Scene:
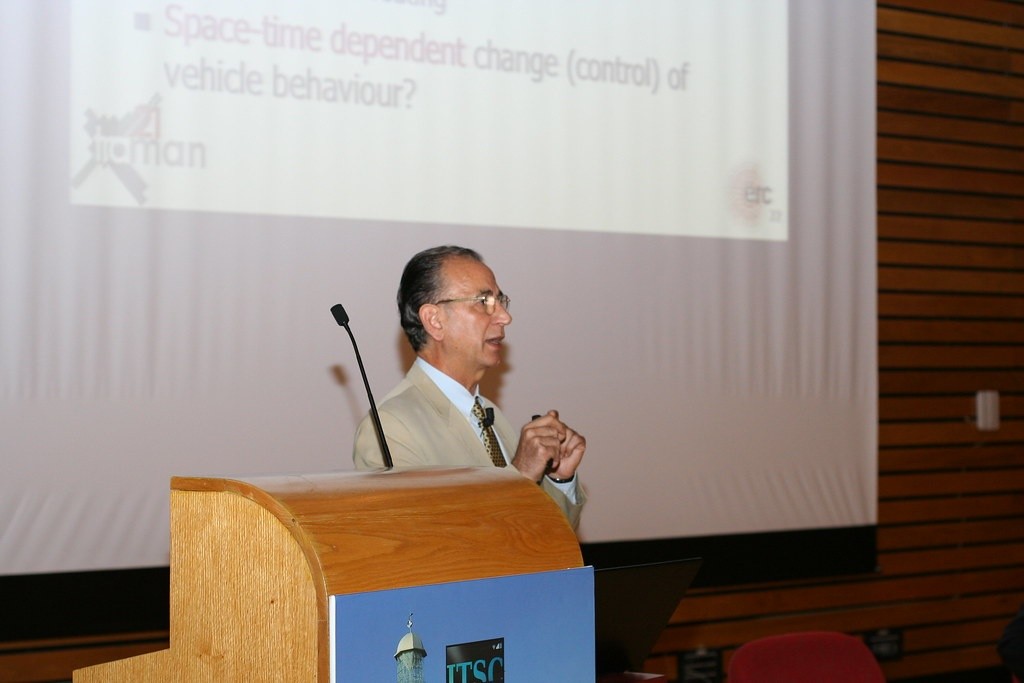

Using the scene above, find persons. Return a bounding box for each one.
[353,242,588,539]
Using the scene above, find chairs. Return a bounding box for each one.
[725,632,888,683]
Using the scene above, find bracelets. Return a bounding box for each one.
[546,473,576,483]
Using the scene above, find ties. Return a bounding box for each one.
[471,398,507,467]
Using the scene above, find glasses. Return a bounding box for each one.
[436,293,512,315]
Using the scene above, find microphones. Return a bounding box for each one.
[329,304,394,470]
[482,408,494,427]
